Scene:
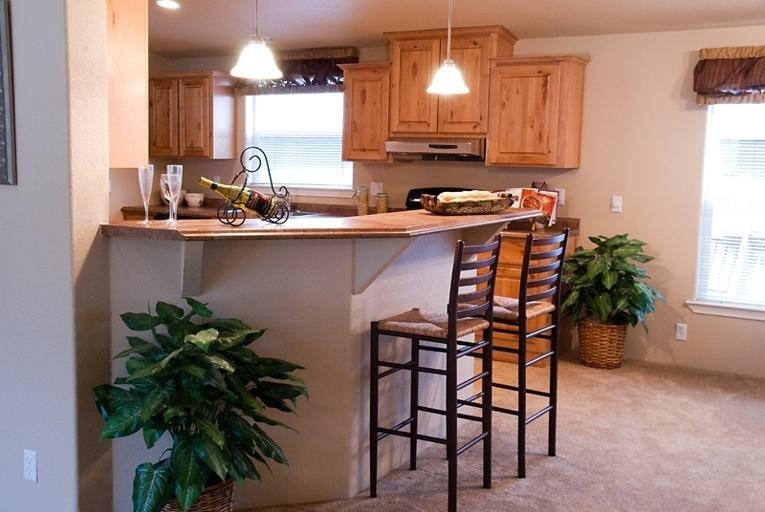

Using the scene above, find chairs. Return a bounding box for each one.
[370,234,502,511]
[447,227,569,478]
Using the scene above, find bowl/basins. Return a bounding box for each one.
[185,193,204,207]
[162,191,185,206]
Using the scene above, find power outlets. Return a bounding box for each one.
[23,449,37,482]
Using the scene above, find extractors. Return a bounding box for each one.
[385,139,489,162]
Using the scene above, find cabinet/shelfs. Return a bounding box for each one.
[486,55,590,169]
[336,61,393,162]
[149,71,236,161]
[476,232,546,369]
[383,25,520,139]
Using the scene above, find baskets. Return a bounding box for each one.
[420,192,514,215]
[577,312,629,370]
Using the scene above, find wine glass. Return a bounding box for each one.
[168,163,182,230]
[160,173,178,226]
[137,165,155,226]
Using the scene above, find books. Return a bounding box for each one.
[494,187,560,227]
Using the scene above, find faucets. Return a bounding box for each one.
[276,192,291,210]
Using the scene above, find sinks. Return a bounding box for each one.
[290,212,312,216]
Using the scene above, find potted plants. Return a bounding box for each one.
[562,234,665,369]
[91,292,309,512]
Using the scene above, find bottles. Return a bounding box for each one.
[356,187,368,217]
[198,176,280,220]
[377,192,389,214]
